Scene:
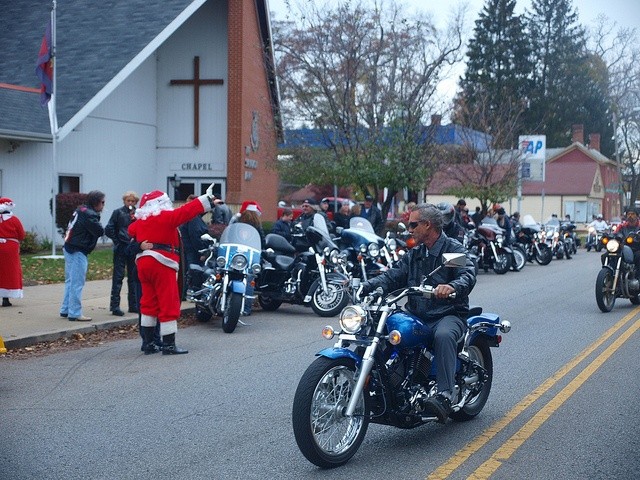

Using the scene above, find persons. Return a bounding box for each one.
[228,200,267,316]
[127,190,217,355]
[104,191,140,316]
[587,213,611,233]
[497,207,520,272]
[334,200,349,228]
[461,208,475,226]
[211,197,232,226]
[124,236,163,351]
[179,194,224,300]
[450,199,467,238]
[401,202,416,222]
[294,198,329,238]
[612,208,640,296]
[472,207,482,226]
[509,212,524,234]
[435,202,465,245]
[320,197,333,221]
[341,203,477,424]
[610,224,617,233]
[486,204,500,220]
[559,214,577,254]
[272,208,293,243]
[0,198,27,307]
[59,191,106,321]
[349,205,361,217]
[592,214,598,221]
[544,213,560,246]
[358,194,385,234]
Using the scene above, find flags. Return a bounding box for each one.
[35,17,54,111]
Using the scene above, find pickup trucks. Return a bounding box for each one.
[277,196,356,221]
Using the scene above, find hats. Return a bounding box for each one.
[237,199,264,218]
[493,204,502,211]
[302,199,316,206]
[626,206,638,216]
[366,195,373,200]
[498,207,505,214]
[0,198,16,212]
[135,190,168,214]
[320,198,330,202]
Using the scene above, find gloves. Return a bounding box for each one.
[206,182,215,196]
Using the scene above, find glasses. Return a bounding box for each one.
[102,201,105,203]
[408,220,424,228]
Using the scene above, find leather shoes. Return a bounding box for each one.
[60,314,68,317]
[69,314,93,321]
[112,310,124,316]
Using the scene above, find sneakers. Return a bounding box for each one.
[426,394,455,424]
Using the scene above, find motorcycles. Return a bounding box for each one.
[333,216,392,284]
[457,215,513,275]
[379,231,411,266]
[292,247,513,469]
[554,221,582,260]
[595,229,639,313]
[542,217,564,261]
[583,224,606,252]
[183,221,263,333]
[513,214,552,265]
[510,242,527,271]
[257,212,351,318]
[396,222,416,249]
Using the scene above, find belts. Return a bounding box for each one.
[152,242,181,255]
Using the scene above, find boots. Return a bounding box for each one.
[144,327,159,355]
[162,333,189,355]
